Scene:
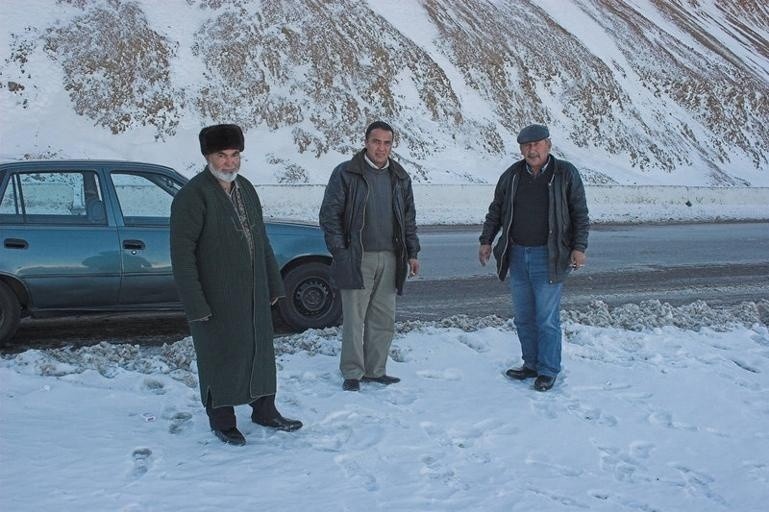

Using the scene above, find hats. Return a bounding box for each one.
[516,123,550,144]
[198,123,245,154]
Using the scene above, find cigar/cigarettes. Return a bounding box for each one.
[569,264,585,268]
[407,272,416,278]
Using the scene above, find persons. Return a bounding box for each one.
[318,121,422,393]
[478,123,592,392]
[168,123,304,448]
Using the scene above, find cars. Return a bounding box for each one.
[0,161,347,345]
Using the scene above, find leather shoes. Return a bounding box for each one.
[342,378,361,392]
[252,411,303,431]
[506,366,537,380]
[211,425,246,446]
[365,374,400,385]
[534,374,557,391]
